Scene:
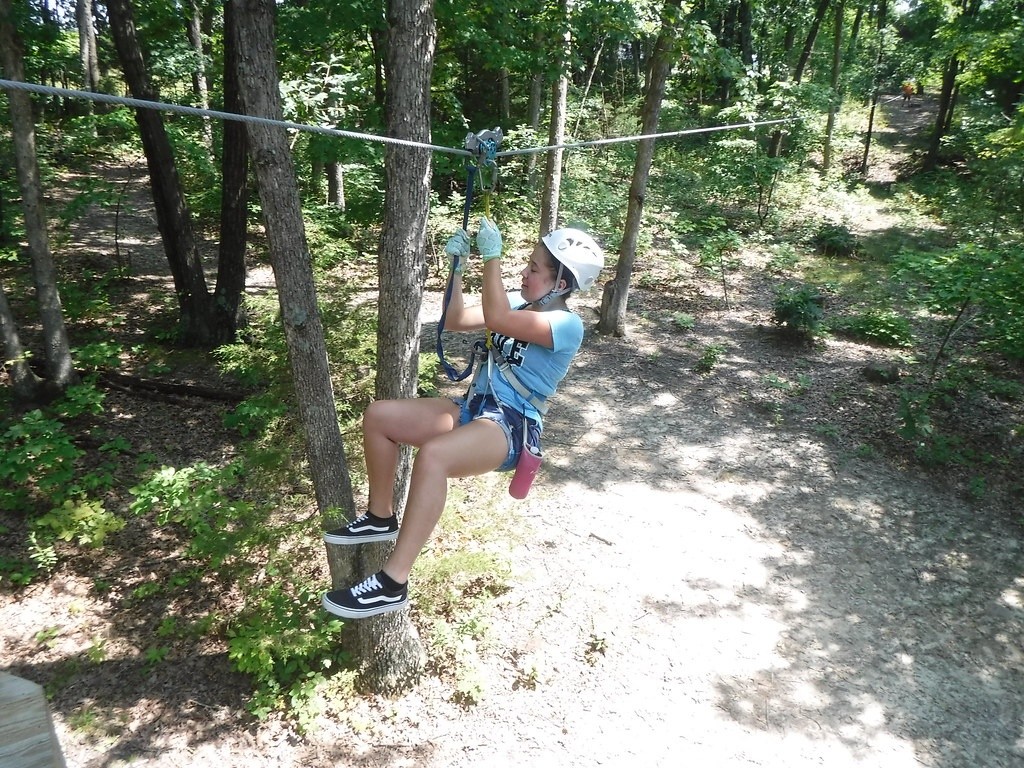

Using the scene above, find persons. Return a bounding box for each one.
[320,216,604,619]
[901,82,913,109]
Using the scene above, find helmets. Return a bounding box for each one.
[542,228,604,291]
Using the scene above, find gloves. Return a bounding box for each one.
[444,229,471,273]
[476,215,502,263]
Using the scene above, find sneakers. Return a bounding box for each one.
[323,511,399,545]
[321,571,408,619]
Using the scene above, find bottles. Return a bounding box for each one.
[529,446,540,456]
